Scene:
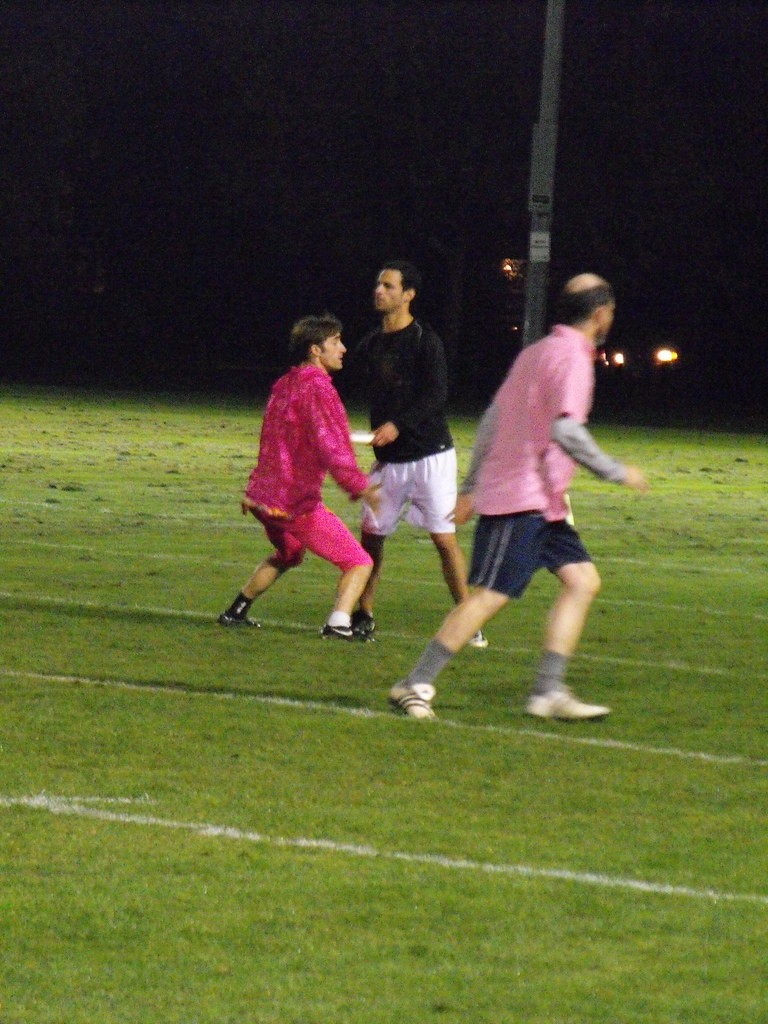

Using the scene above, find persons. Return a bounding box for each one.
[388,272,652,720]
[215,259,490,650]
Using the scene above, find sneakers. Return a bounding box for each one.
[526,682,612,719]
[319,624,371,644]
[389,682,437,722]
[216,609,262,628]
[351,609,376,635]
[470,630,490,648]
